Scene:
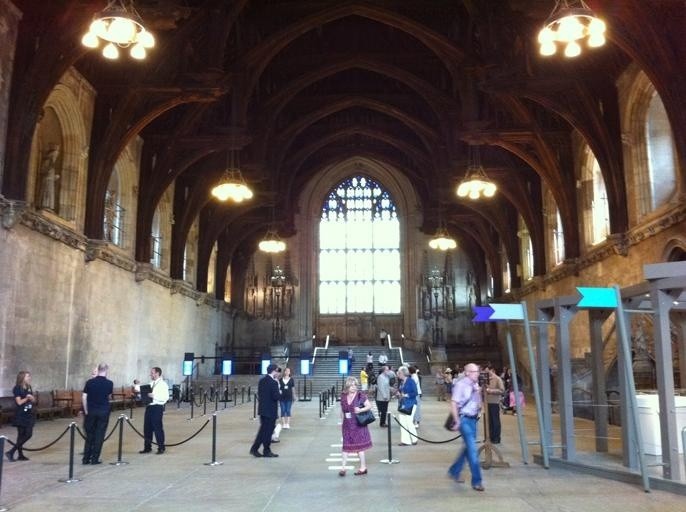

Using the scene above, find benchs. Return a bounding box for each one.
[0,383,144,422]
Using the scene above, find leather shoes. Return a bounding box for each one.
[398,442,411,446]
[411,442,418,445]
[338,469,346,476]
[154,447,166,456]
[262,452,280,457]
[353,467,369,477]
[414,424,419,430]
[472,483,485,492]
[80,457,104,465]
[446,471,466,484]
[138,448,152,453]
[248,447,263,458]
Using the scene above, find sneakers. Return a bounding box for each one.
[270,438,281,443]
[16,456,29,462]
[379,423,389,428]
[4,450,16,463]
[281,423,290,429]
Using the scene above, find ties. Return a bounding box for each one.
[151,381,156,390]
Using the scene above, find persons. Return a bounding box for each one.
[432,364,523,417]
[448,362,486,493]
[132,379,145,407]
[346,347,353,371]
[269,367,283,443]
[3,371,37,463]
[379,328,387,346]
[81,363,112,466]
[278,367,296,430]
[481,362,506,443]
[137,366,168,455]
[249,364,283,458]
[337,375,372,477]
[359,350,422,447]
[79,367,98,455]
[135,380,141,399]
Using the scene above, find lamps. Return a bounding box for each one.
[453,138,498,199]
[429,198,457,252]
[534,0,610,58]
[259,191,287,254]
[79,0,155,62]
[208,83,255,203]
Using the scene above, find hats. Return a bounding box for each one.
[445,367,453,372]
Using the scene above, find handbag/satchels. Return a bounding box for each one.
[396,395,415,416]
[354,391,376,426]
[443,406,465,432]
